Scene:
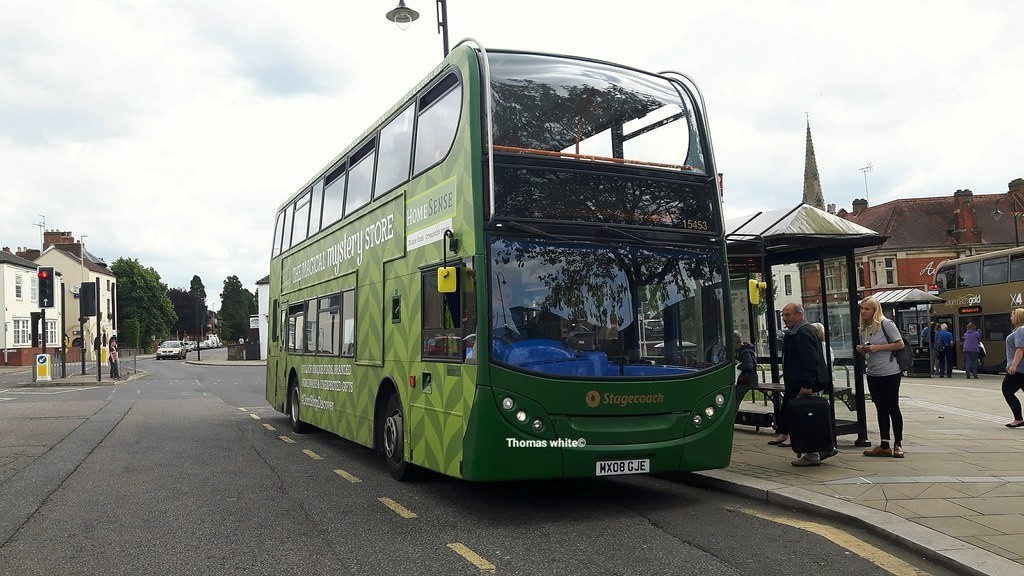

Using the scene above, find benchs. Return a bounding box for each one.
[751,365,855,412]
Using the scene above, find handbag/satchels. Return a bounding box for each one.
[935,338,945,352]
[733,362,742,387]
[978,342,987,357]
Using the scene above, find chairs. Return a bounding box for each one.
[514,143,561,158]
[536,307,564,343]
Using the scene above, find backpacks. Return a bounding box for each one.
[880,320,913,370]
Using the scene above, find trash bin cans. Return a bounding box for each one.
[246,340,259,360]
[907,347,931,378]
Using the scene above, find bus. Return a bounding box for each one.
[265,37,738,484]
[931,246,1024,374]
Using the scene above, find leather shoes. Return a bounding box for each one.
[863,446,892,456]
[892,445,904,458]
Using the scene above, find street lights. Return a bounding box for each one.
[386,0,449,59]
[990,197,1019,247]
[81,235,88,282]
[195,299,200,360]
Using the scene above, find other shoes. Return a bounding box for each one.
[791,454,821,467]
[947,375,952,378]
[939,374,944,378]
[1005,420,1024,427]
[768,437,791,447]
[821,447,839,460]
[974,376,979,379]
[967,376,970,379]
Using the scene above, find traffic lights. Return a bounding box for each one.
[212,303,216,333]
[38,267,53,307]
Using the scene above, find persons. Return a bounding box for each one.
[1002,308,1024,428]
[491,283,519,335]
[963,322,981,379]
[767,322,835,447]
[109,339,120,380]
[856,297,906,458]
[782,303,839,467]
[920,321,955,378]
[733,330,758,421]
[238,336,244,345]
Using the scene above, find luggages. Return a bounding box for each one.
[790,395,838,458]
